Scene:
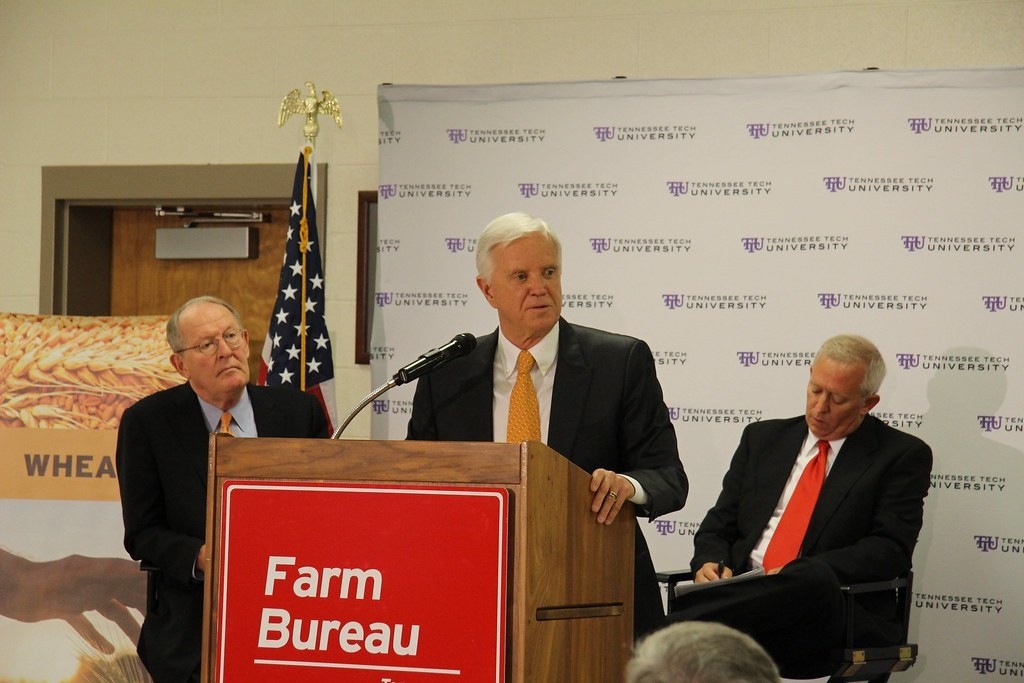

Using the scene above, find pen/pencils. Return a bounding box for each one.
[718,564,721,579]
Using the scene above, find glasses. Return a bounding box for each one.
[176,328,243,356]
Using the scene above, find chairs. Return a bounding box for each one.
[656,567,918,683]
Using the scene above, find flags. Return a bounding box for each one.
[255,142,338,436]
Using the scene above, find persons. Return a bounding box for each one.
[690,335,933,585]
[116,296,331,683]
[405,212,690,643]
[626,621,778,683]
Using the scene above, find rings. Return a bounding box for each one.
[609,491,617,499]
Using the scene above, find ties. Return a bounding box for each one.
[219,412,233,433]
[762,440,830,576]
[506,351,541,442]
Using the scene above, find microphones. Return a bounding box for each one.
[398,333,477,383]
